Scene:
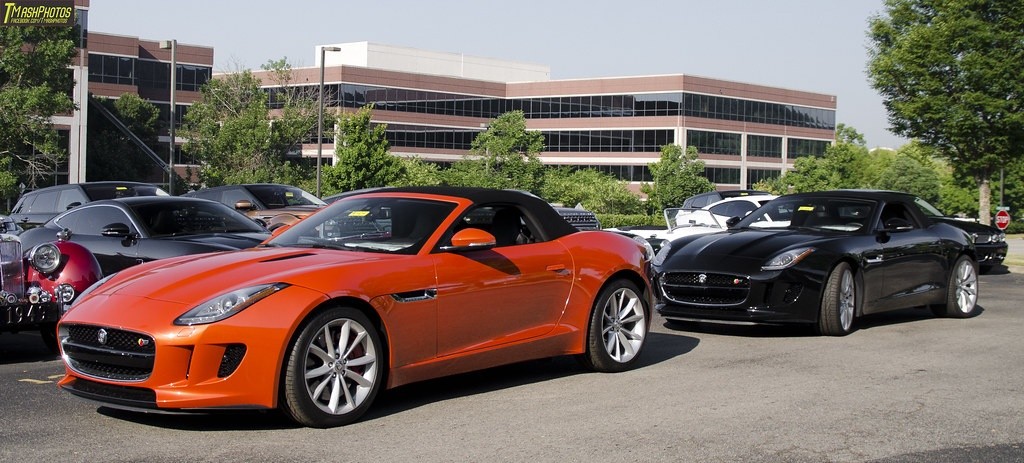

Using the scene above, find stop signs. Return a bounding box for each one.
[995,210,1010,230]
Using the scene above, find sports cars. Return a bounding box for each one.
[59,186,652,428]
[650,189,981,336]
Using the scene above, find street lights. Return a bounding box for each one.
[160,39,178,195]
[317,46,341,198]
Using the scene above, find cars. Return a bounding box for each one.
[912,196,1008,274]
[178,182,330,232]
[676,195,791,227]
[0,217,106,354]
[603,207,729,254]
[12,181,171,237]
[551,203,600,234]
[677,190,775,216]
[18,195,272,277]
